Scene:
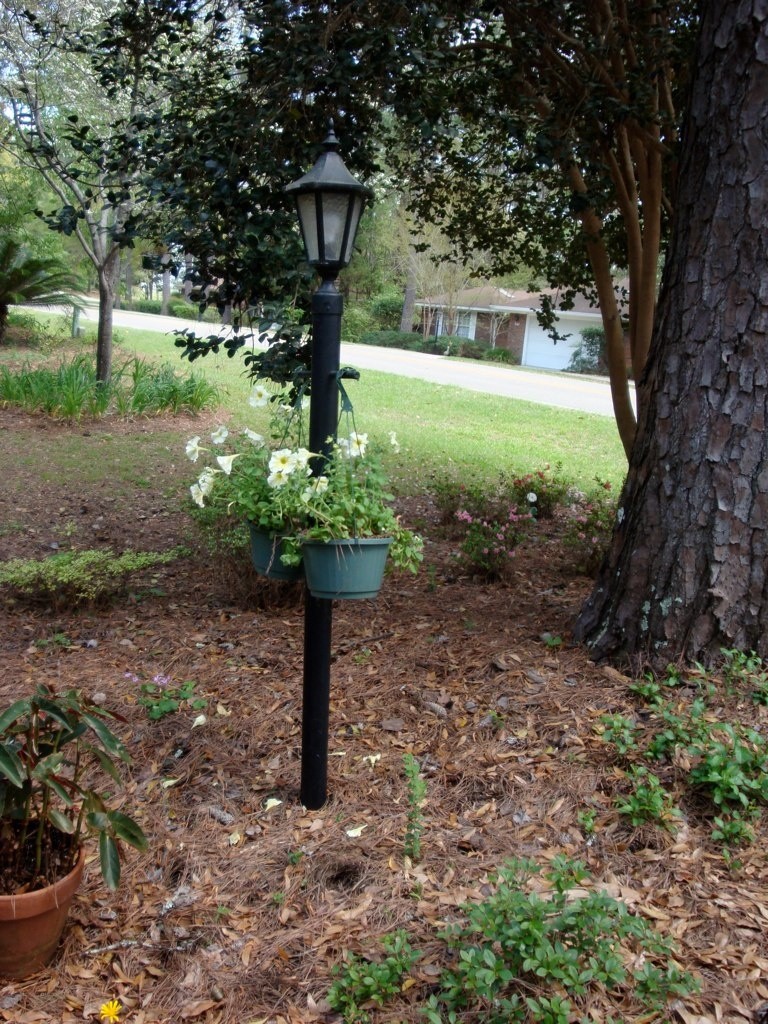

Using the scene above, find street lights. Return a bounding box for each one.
[279,128,375,811]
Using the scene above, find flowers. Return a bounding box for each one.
[186,390,313,534]
[269,433,424,575]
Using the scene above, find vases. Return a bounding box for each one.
[240,516,299,580]
[299,537,393,599]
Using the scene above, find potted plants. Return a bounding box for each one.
[0,680,148,978]
